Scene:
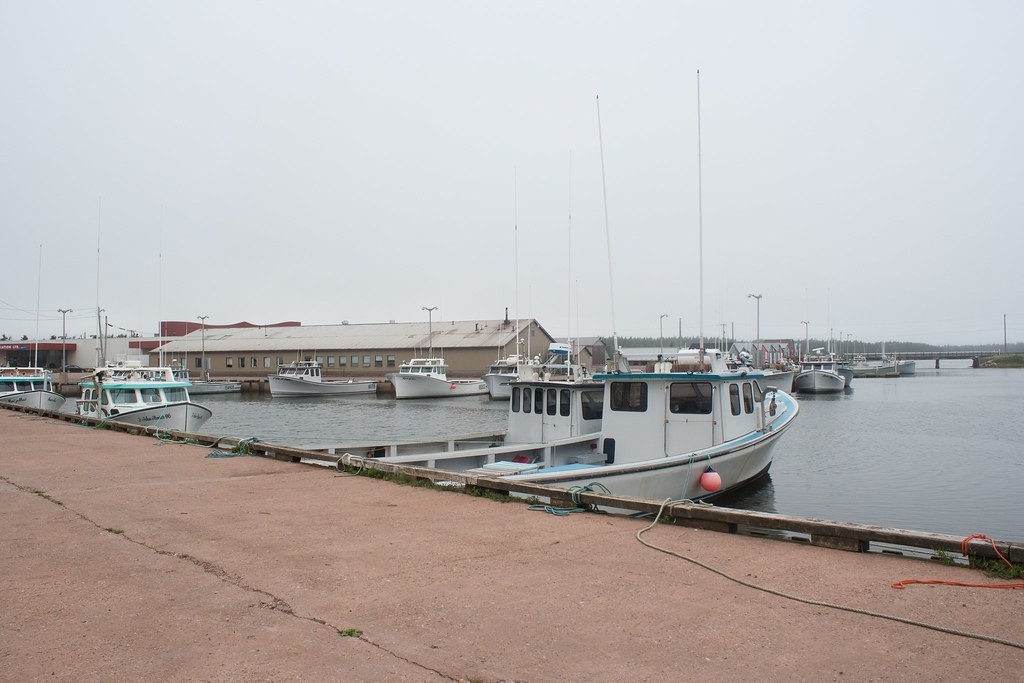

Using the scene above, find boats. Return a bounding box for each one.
[298,370,800,518]
[386,341,489,400]
[267,341,377,399]
[0,189,244,437]
[481,66,915,401]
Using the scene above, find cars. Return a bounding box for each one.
[60,364,86,373]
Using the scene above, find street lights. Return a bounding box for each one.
[748,293,762,369]
[660,314,668,353]
[57,308,73,373]
[800,321,811,354]
[197,315,208,369]
[421,307,438,358]
